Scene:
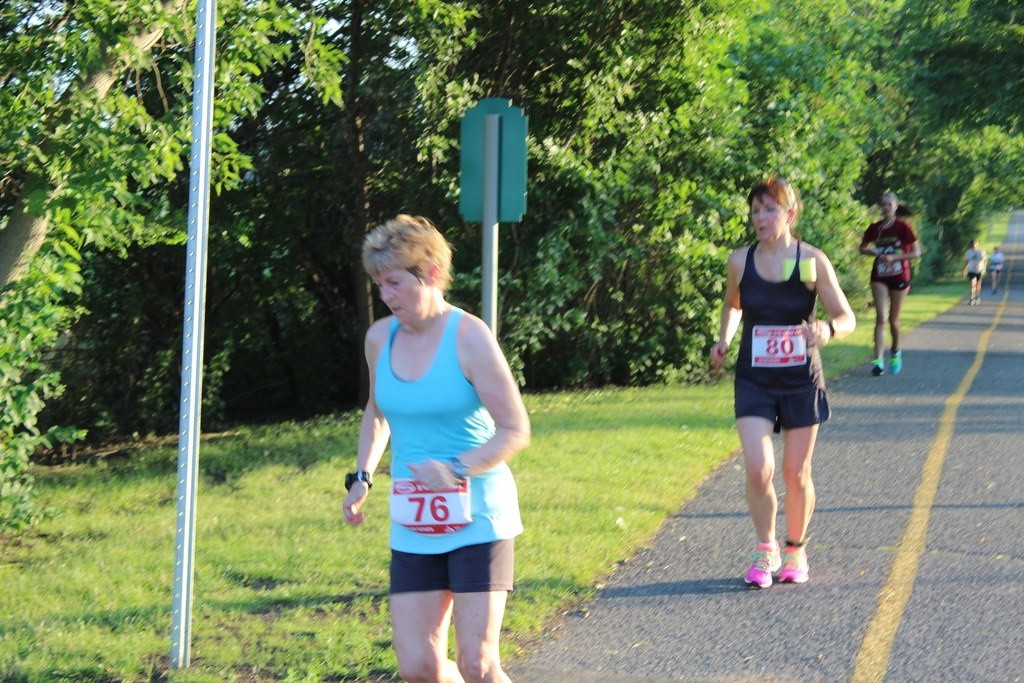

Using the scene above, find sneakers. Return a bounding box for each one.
[744,543,781,590]
[889,350,903,375]
[778,546,811,586]
[871,356,885,377]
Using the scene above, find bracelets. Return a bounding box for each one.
[447,456,469,478]
[709,174,857,594]
[827,319,837,338]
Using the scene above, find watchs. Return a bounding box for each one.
[344,470,375,493]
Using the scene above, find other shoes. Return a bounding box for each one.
[968,296,980,306]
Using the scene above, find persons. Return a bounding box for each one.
[855,188,925,379]
[342,213,533,683]
[988,245,1006,296]
[961,237,985,307]
